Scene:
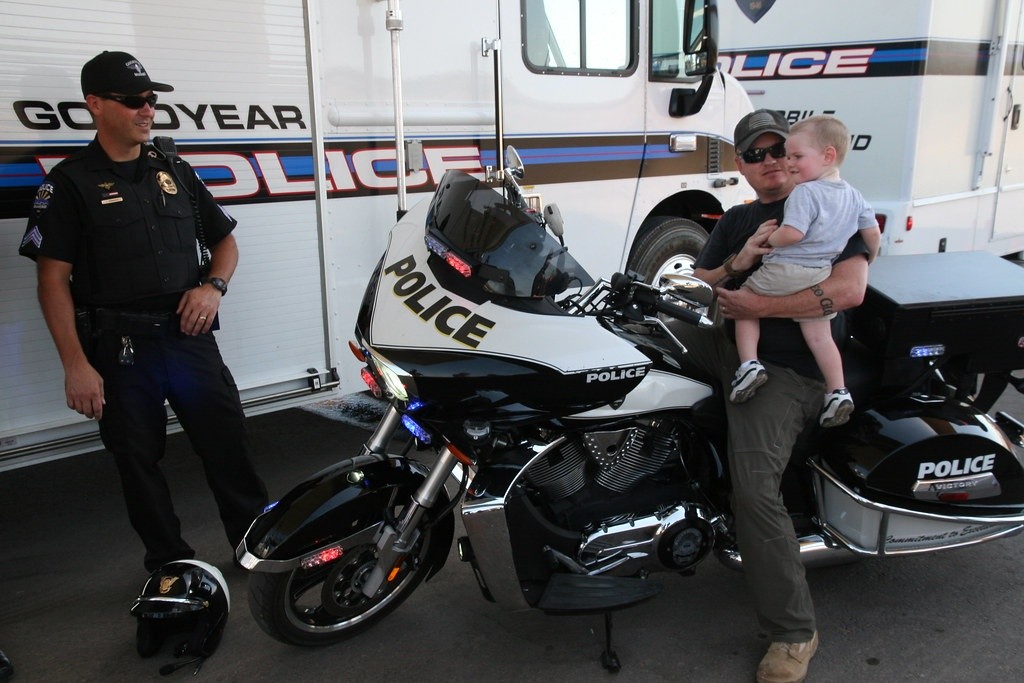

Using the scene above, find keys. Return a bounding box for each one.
[118,335,136,367]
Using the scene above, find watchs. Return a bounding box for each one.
[722,252,742,277]
[201,276,227,297]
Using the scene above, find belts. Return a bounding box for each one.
[75,304,179,337]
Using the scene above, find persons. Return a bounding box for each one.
[690,109,881,683]
[18,50,271,573]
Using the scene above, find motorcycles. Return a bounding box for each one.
[236,147,1024,676]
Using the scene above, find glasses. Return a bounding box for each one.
[98,94,157,109]
[736,141,786,164]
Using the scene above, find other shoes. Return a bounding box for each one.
[756,630,818,683]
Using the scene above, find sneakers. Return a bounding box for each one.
[819,387,854,427]
[728,360,768,403]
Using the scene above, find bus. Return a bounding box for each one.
[651,1,1024,279]
[0,2,772,474]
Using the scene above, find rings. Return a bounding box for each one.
[198,316,207,320]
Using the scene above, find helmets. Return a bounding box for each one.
[130,558,230,659]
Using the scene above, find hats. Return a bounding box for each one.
[734,108,790,155]
[81,50,174,100]
